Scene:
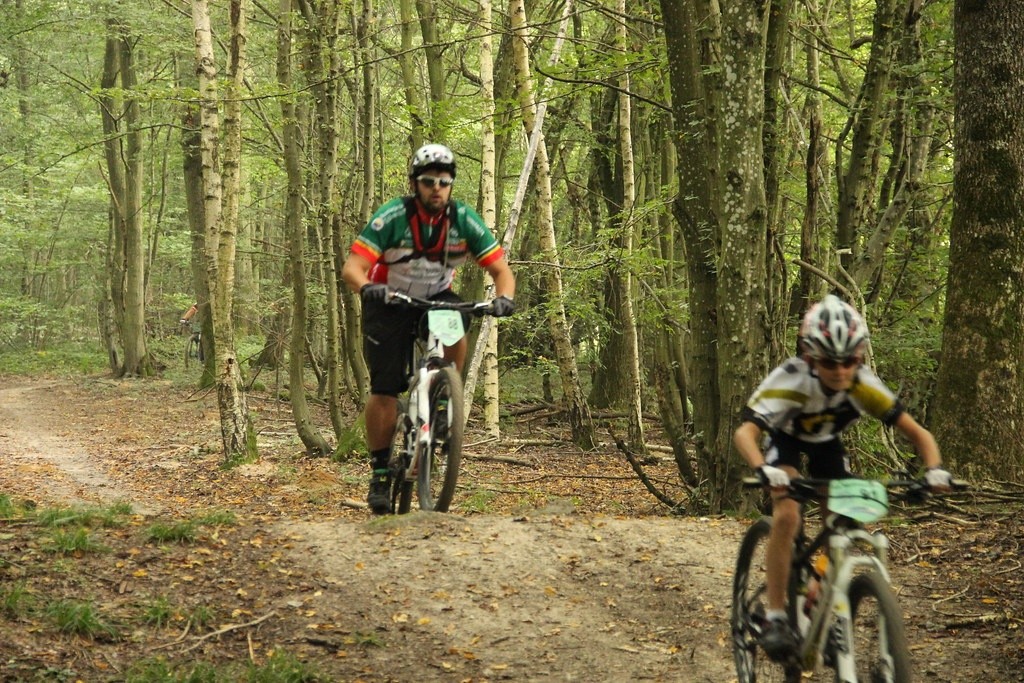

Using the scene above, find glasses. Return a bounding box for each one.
[417,175,453,188]
[813,357,860,370]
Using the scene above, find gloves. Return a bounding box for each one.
[925,469,951,492]
[492,296,516,317]
[360,284,390,305]
[755,465,790,487]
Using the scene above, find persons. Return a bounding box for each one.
[342,143,516,513]
[732,294,951,656]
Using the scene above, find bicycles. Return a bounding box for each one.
[388,290,496,514]
[730,476,971,683]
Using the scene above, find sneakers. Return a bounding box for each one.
[435,400,448,439]
[367,467,391,513]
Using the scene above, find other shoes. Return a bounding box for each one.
[763,611,798,662]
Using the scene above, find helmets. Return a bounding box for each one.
[797,288,869,360]
[409,144,457,178]
